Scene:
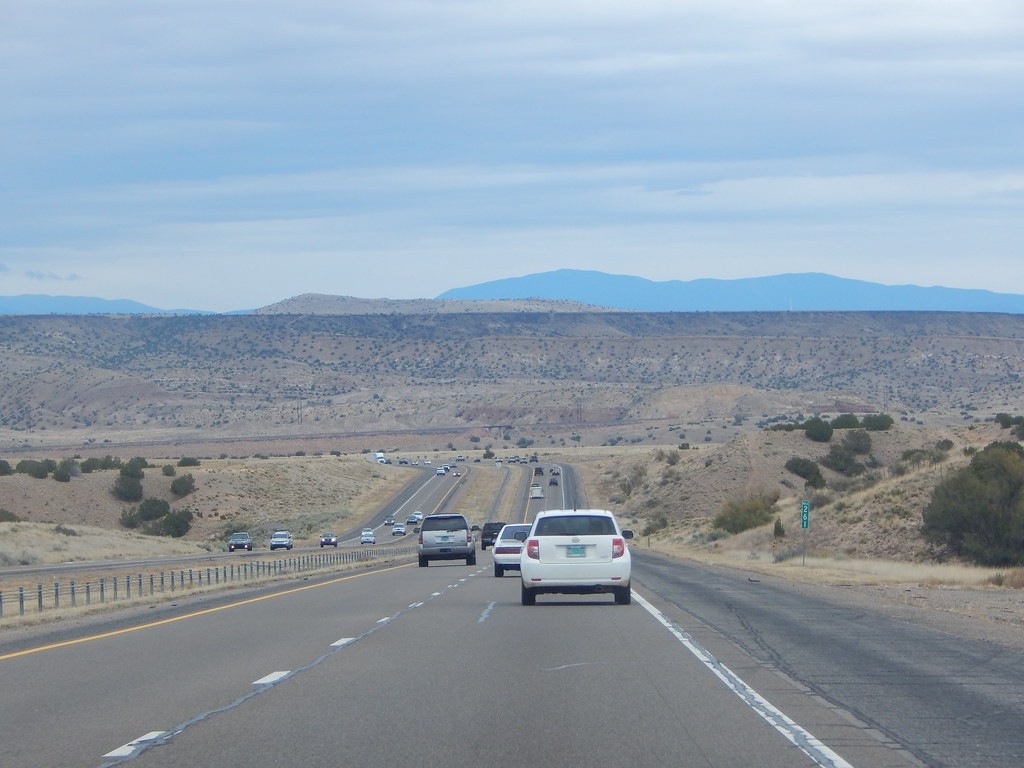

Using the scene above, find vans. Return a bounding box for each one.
[529,486,544,499]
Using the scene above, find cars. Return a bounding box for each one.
[384,458,392,463]
[384,509,423,537]
[436,455,466,476]
[359,526,377,546]
[534,467,545,476]
[494,454,538,464]
[548,465,561,487]
[423,459,432,465]
[472,456,483,462]
[228,531,256,551]
[319,531,337,546]
[411,459,419,465]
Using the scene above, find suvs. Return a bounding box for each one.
[399,457,408,465]
[481,521,533,577]
[513,509,633,607]
[416,512,480,567]
[270,529,294,550]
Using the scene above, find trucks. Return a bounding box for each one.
[373,453,386,463]
